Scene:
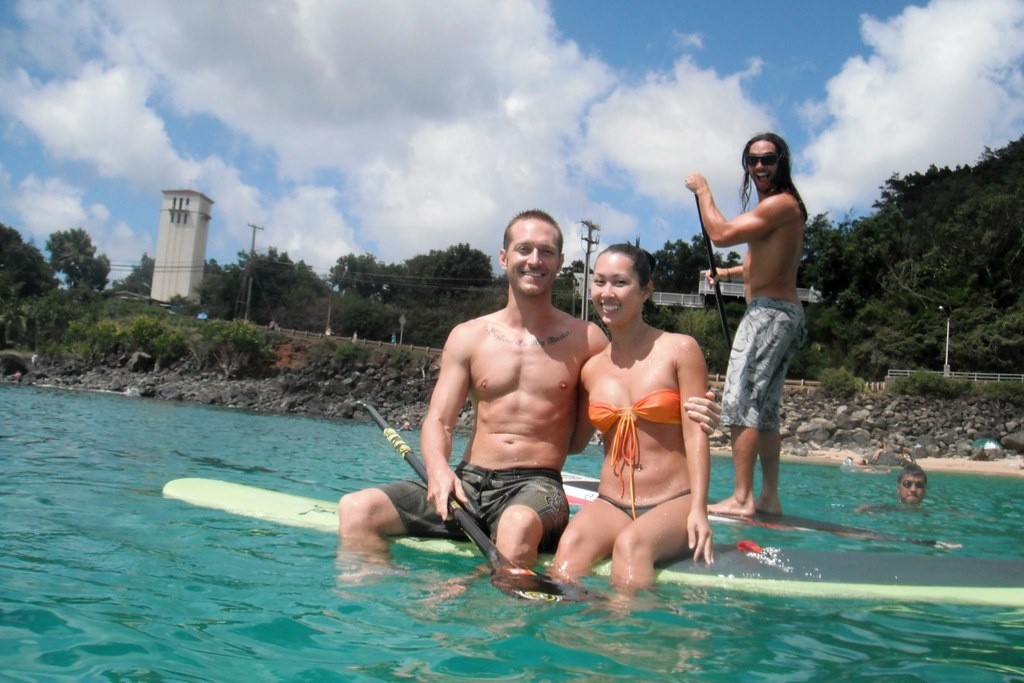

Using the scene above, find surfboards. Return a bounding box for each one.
[559,470,963,550]
[159,475,1023,610]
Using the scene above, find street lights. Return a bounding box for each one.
[938,305,950,377]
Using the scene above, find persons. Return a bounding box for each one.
[338,211,721,583]
[683,132,808,517]
[897,464,927,504]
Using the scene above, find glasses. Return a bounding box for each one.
[745,154,779,167]
[899,480,925,489]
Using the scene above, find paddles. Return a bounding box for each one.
[693,191,733,349]
[359,400,523,568]
